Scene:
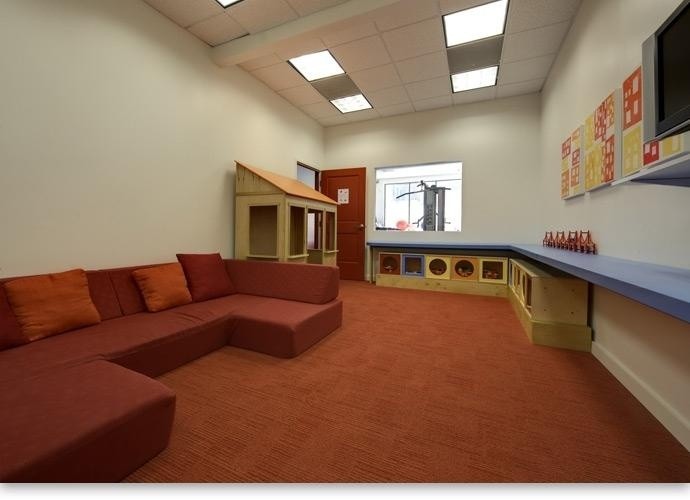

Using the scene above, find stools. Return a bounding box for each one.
[1,356,179,483]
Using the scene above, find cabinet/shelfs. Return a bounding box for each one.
[373,249,509,301]
[504,255,594,355]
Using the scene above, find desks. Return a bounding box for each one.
[364,237,690,327]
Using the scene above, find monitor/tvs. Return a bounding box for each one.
[641,0,690,145]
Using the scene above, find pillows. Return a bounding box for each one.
[130,262,192,314]
[4,266,101,345]
[175,251,242,304]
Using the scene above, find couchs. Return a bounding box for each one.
[0,256,345,382]
